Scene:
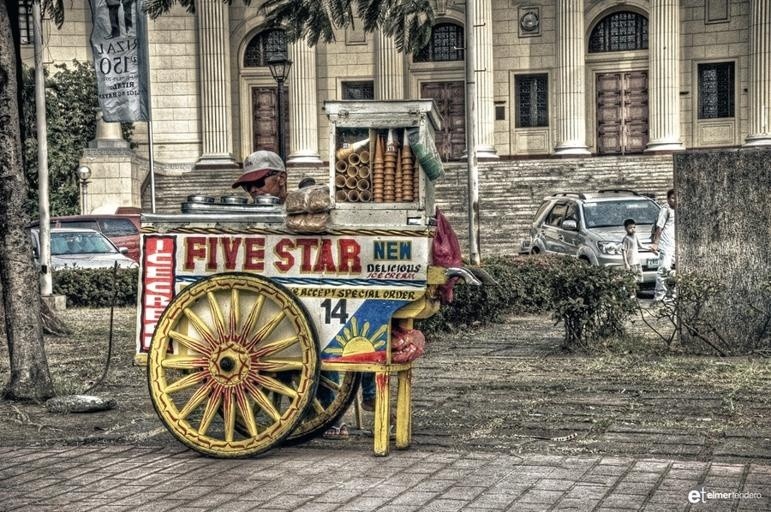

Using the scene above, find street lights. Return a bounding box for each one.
[266,48,295,194]
[75,165,92,217]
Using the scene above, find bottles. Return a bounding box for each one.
[410,140,441,179]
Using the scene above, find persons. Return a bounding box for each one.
[621,219,652,306]
[232,150,396,440]
[652,188,675,307]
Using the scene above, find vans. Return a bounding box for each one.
[28,214,141,265]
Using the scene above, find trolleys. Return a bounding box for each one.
[137,95,445,459]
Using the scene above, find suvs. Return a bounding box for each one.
[529,185,680,299]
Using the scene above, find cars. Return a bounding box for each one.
[27,226,140,273]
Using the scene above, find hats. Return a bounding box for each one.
[232,149,286,192]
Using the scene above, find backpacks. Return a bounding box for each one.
[649,210,669,242]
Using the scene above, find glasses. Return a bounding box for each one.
[241,176,271,192]
